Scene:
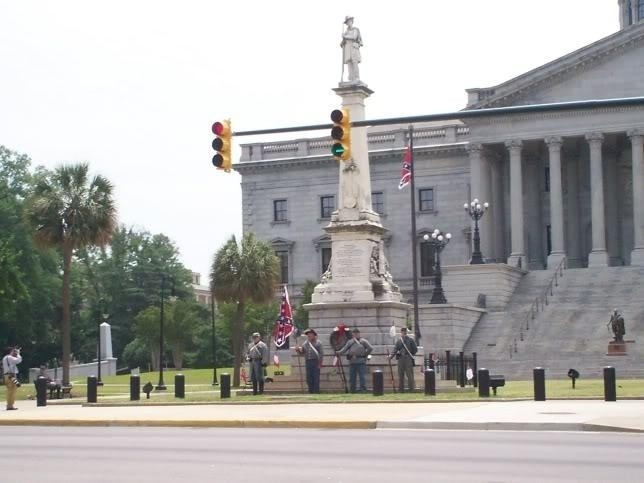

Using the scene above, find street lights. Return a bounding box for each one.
[209,272,219,385]
[423,229,452,305]
[463,197,489,265]
[155,276,179,390]
[96,297,110,386]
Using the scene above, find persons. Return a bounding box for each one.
[387,327,418,392]
[606,308,625,344]
[2,347,22,410]
[340,16,364,82]
[245,333,268,395]
[33,363,62,399]
[295,328,324,393]
[335,328,374,393]
[432,352,441,373]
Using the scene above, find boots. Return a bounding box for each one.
[253,380,257,394]
[258,382,263,393]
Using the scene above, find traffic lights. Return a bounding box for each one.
[211,121,232,171]
[330,108,352,161]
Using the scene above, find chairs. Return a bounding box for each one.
[38,375,55,399]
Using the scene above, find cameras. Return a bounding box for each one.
[14,348,17,353]
[8,375,21,387]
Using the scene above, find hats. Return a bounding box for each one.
[352,329,360,333]
[253,332,260,336]
[305,329,317,336]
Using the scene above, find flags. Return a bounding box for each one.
[274,289,294,348]
[398,139,412,190]
